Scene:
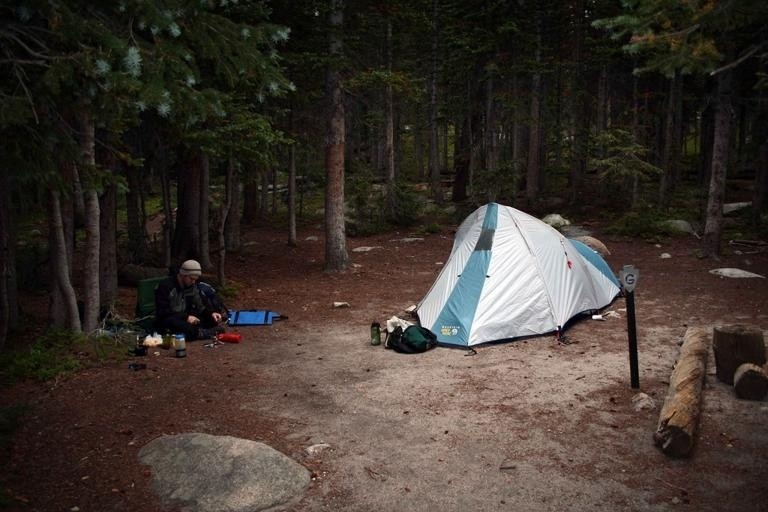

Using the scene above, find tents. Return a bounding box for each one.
[404,199,620,350]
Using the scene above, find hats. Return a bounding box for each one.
[180,259,201,276]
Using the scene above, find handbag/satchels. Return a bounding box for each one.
[400,325,438,352]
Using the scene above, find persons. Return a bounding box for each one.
[154,260,232,343]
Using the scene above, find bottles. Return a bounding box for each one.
[370,319,381,346]
[162,332,186,358]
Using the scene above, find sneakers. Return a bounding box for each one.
[205,326,225,336]
